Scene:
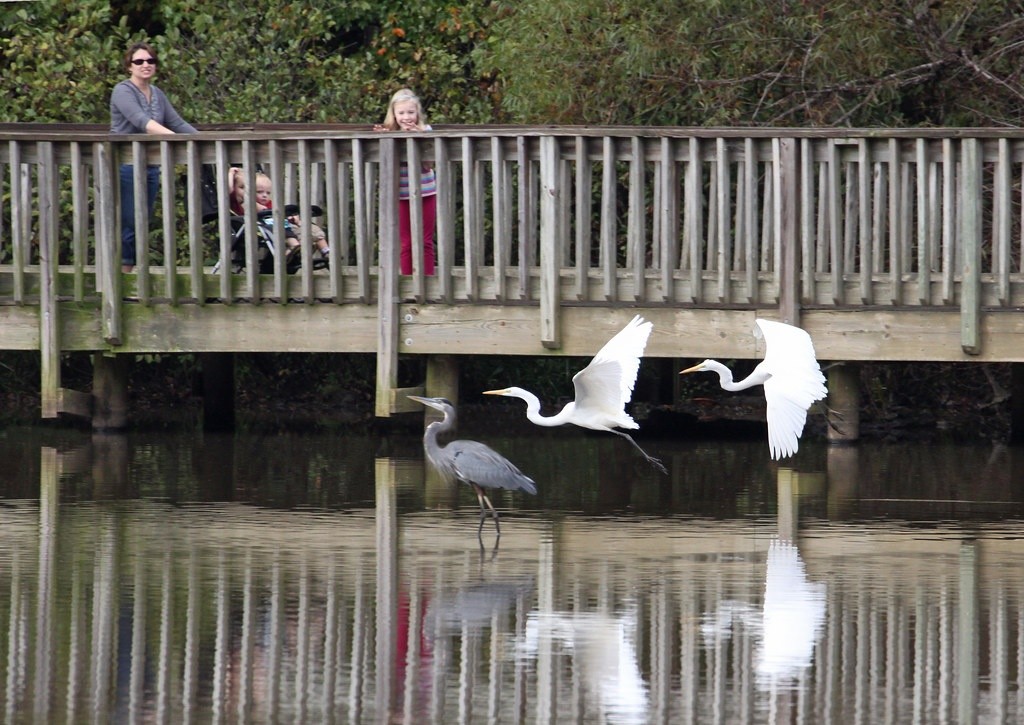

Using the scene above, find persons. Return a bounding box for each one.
[253,169,336,266]
[227,165,306,268]
[374,87,439,277]
[108,40,202,304]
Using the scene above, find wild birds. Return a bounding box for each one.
[403,390,538,536]
[481,312,668,479]
[677,318,848,461]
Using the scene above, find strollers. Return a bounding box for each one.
[185,163,325,275]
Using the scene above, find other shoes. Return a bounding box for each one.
[322,250,329,258]
[286,245,301,266]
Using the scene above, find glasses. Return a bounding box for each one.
[130,58,155,65]
